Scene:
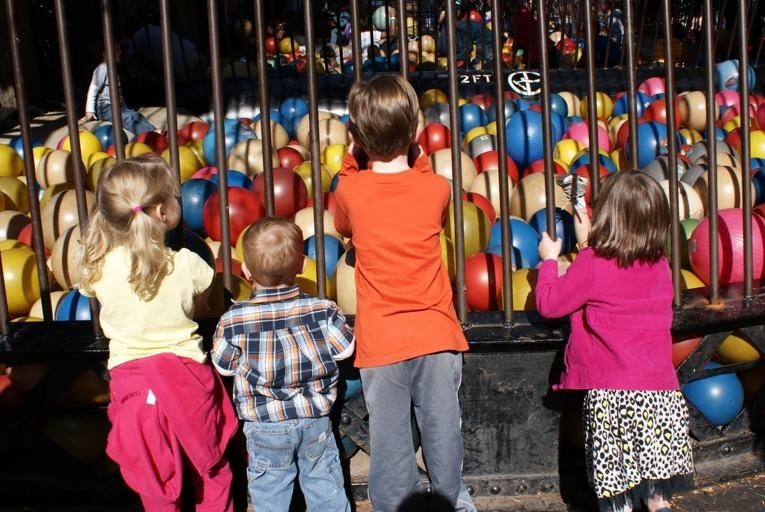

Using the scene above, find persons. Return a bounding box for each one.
[211,219,357,512]
[264,2,765,93]
[536,170,695,512]
[79,159,239,512]
[77,36,168,135]
[334,74,474,512]
